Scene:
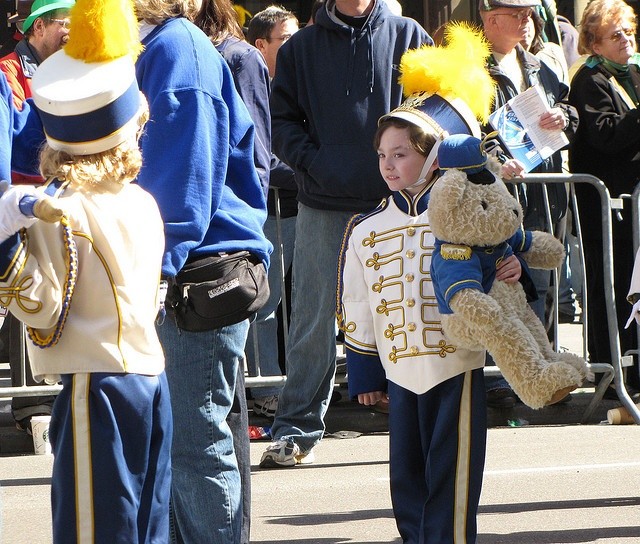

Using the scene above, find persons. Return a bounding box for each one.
[0,0,76,197]
[519,10,570,105]
[570,0,640,402]
[131,0,275,543]
[245,4,299,419]
[336,19,522,544]
[194,0,272,202]
[259,0,436,466]
[0,0,173,544]
[478,0,580,330]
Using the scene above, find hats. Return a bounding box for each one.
[378,90,498,190]
[29,46,149,156]
[478,0,541,11]
[7,1,33,23]
[22,0,76,32]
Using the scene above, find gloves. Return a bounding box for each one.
[624,300,640,329]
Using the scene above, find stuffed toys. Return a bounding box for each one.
[428,133,587,411]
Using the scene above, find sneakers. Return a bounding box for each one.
[259,436,315,468]
[15,413,51,436]
[252,395,278,422]
[485,388,517,408]
[351,395,389,414]
[595,384,640,404]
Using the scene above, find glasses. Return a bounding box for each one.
[50,18,71,30]
[494,8,532,20]
[602,28,634,42]
[261,35,291,41]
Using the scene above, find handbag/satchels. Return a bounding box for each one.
[164,250,271,332]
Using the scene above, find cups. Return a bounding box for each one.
[606,403,640,424]
